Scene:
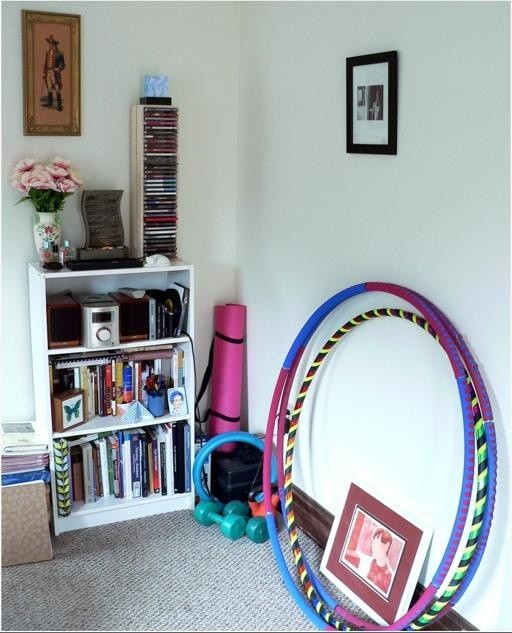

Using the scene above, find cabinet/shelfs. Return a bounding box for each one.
[28,262,196,538]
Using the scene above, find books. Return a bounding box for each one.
[142,107,179,261]
[2,343,195,517]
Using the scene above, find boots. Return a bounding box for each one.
[57,94,63,111]
[42,93,52,107]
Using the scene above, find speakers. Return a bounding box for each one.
[46,294,149,349]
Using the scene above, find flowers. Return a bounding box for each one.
[10,156,83,211]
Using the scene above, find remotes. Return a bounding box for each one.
[117,286,146,299]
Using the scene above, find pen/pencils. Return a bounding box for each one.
[146,367,167,390]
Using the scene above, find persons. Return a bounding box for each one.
[369,85,383,119]
[41,34,67,114]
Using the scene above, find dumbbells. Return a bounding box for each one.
[222,499,270,544]
[191,499,247,542]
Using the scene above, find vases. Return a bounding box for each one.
[34,212,61,264]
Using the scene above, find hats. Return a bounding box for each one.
[45,34,59,45]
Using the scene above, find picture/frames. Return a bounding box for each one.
[318,476,435,626]
[346,50,399,153]
[21,8,81,137]
